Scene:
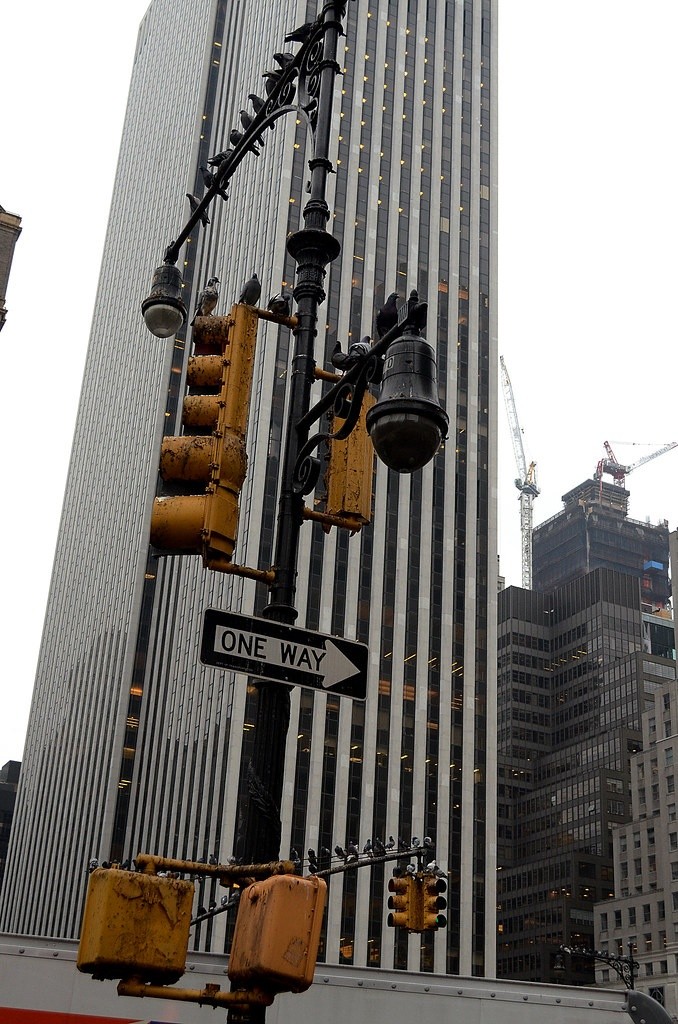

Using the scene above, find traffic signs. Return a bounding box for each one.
[195,607,371,703]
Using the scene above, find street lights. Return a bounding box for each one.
[74,2,457,1023]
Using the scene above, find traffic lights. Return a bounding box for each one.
[423,877,454,933]
[316,387,377,533]
[384,876,410,935]
[146,301,260,578]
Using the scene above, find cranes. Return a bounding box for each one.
[594,440,678,491]
[496,356,535,589]
